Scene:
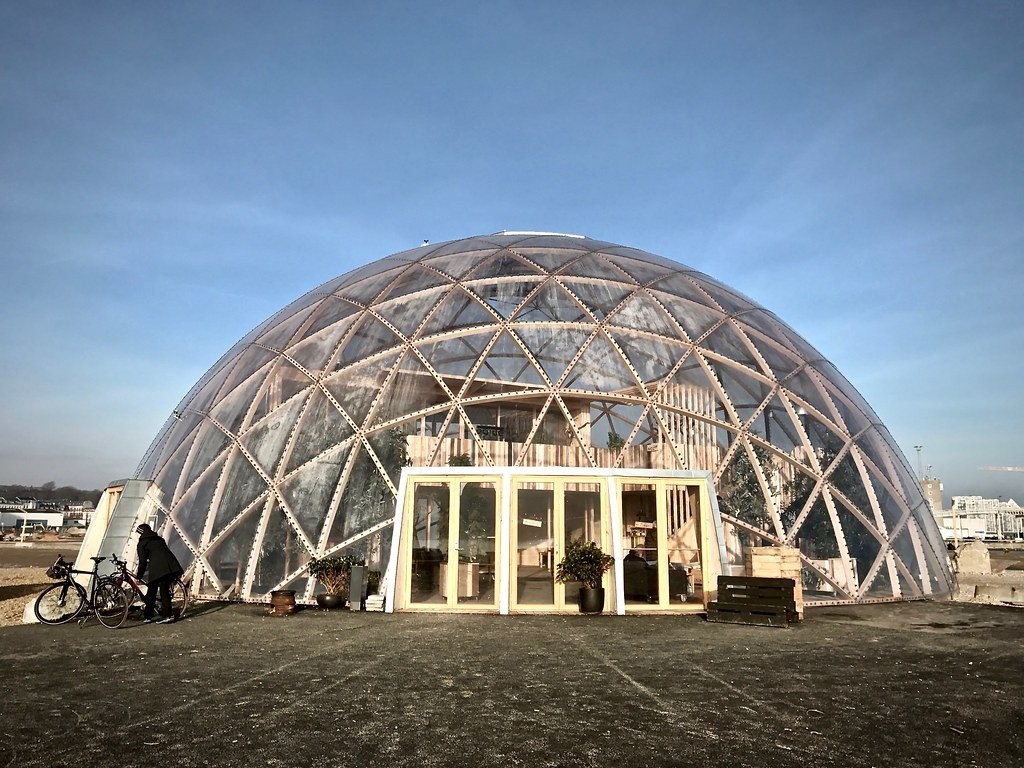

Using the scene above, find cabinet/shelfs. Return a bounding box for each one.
[440,562,480,602]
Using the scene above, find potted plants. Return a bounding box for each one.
[307,554,364,610]
[554,539,616,613]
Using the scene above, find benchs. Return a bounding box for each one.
[802,558,859,596]
[705,576,799,628]
[412,547,443,571]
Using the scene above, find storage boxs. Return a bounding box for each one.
[745,545,804,620]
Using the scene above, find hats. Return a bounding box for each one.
[135,524,151,534]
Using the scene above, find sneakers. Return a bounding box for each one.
[156,616,171,624]
[141,618,152,623]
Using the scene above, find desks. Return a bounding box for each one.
[478,564,492,588]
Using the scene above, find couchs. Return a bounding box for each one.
[624,566,686,604]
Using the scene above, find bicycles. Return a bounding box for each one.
[98,553,188,619]
[34,553,128,629]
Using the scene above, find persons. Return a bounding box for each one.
[10,526,20,540]
[625,544,648,566]
[136,523,184,624]
[948,540,959,572]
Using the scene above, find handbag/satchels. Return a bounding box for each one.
[45,556,66,580]
